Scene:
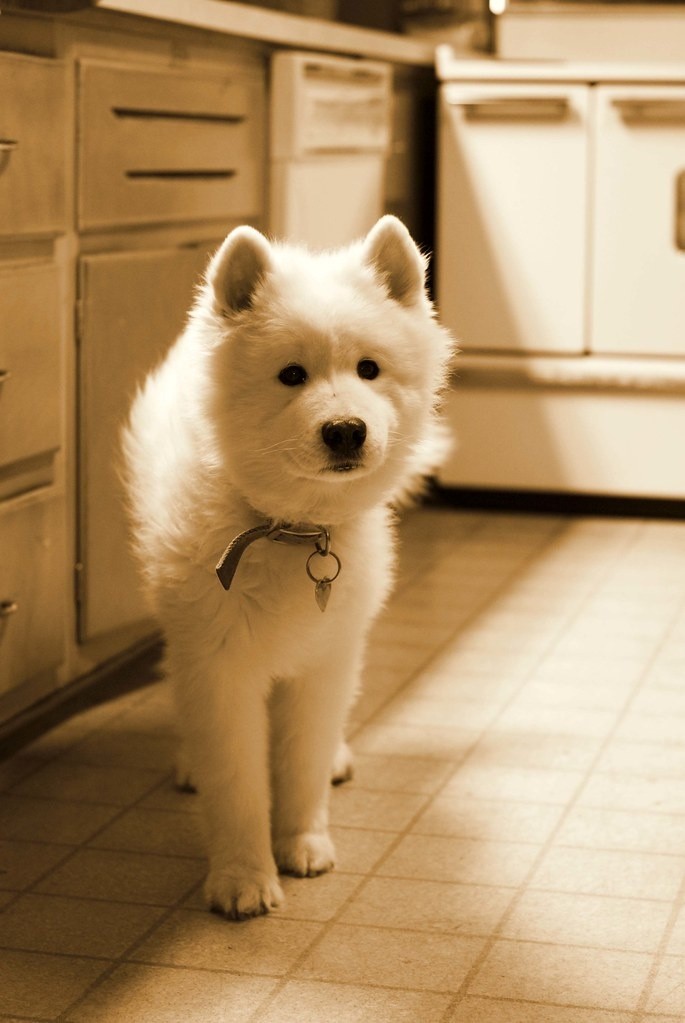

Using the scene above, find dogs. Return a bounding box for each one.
[111,214,460,922]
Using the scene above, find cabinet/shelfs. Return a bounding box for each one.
[77,38,285,670]
[1,21,74,694]
